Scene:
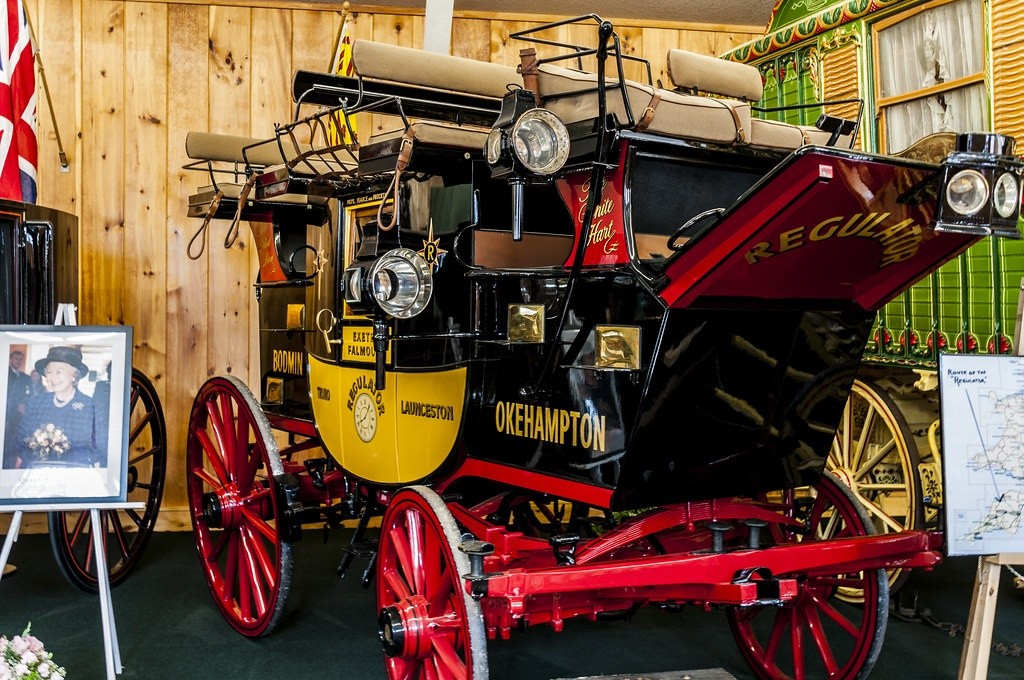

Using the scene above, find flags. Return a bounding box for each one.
[0,0,41,206]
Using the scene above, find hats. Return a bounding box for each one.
[34,346,89,380]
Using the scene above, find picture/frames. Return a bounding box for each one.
[0,324,135,503]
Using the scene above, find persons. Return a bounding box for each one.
[5,346,112,469]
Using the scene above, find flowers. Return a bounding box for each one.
[0,620,66,680]
